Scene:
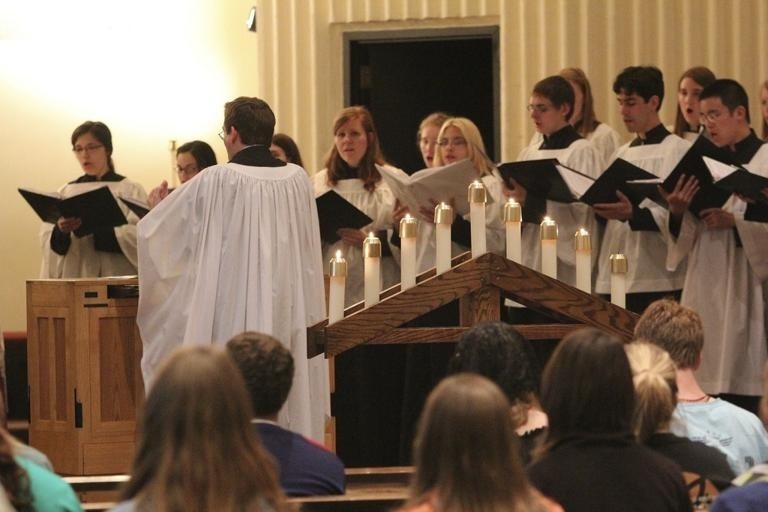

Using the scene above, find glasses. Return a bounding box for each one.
[699,112,725,125]
[524,103,550,114]
[435,137,467,148]
[72,142,103,154]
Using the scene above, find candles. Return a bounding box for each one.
[503,198,523,264]
[328,249,348,325]
[610,252,628,308]
[541,215,558,279]
[434,202,454,275]
[468,178,486,258]
[399,213,418,291]
[362,232,382,306]
[575,227,592,294]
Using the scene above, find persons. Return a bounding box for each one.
[1,399,86,512]
[105,343,300,512]
[225,332,346,498]
[391,300,768,512]
[40,65,768,448]
